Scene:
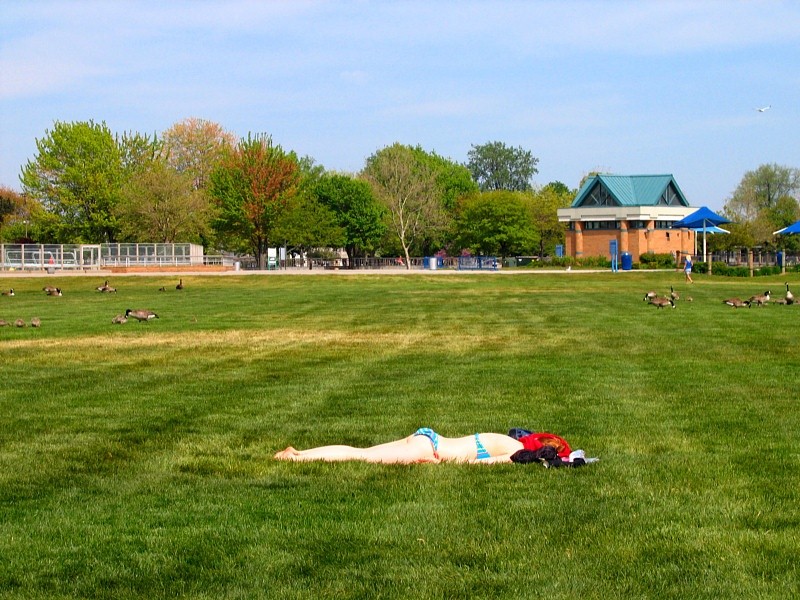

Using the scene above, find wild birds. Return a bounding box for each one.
[0,278,184,326]
[643,284,691,310]
[757,105,771,112]
[723,282,800,309]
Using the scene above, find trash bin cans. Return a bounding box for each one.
[621,254,632,270]
[429,258,438,270]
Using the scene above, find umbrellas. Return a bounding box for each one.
[671,206,732,263]
[773,220,800,237]
[690,225,731,257]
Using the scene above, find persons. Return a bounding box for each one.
[684,255,693,284]
[395,255,405,266]
[274,427,573,468]
[674,249,681,271]
